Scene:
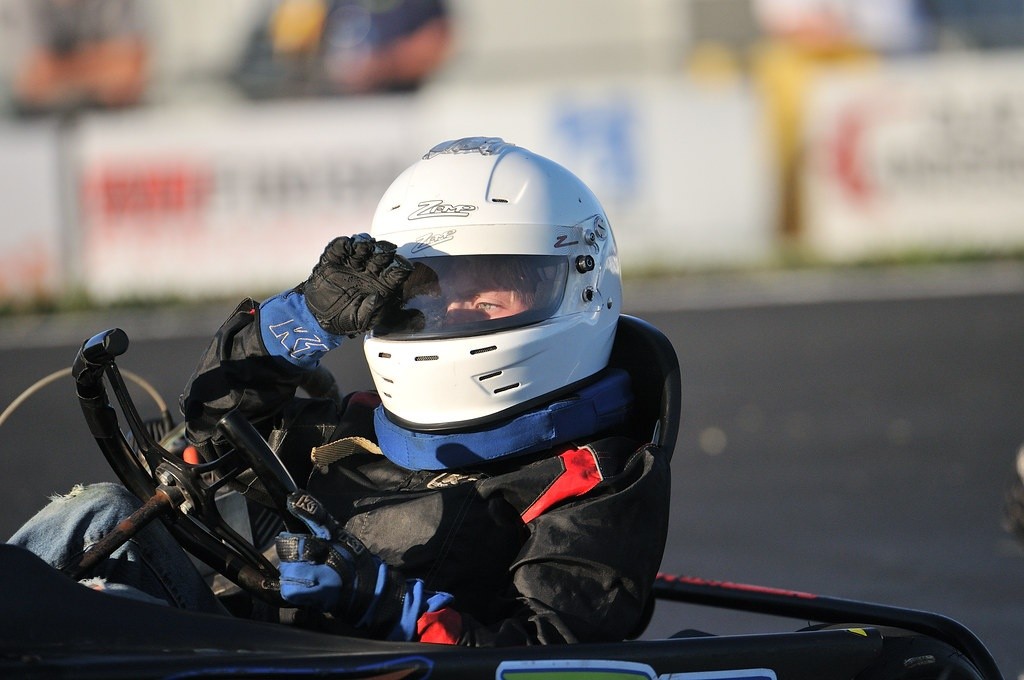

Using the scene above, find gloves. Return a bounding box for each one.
[260,233,412,367]
[277,489,455,641]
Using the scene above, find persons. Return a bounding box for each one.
[266,0,447,93]
[16,0,141,117]
[6,134,682,648]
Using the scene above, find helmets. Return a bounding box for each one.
[361,136,625,435]
